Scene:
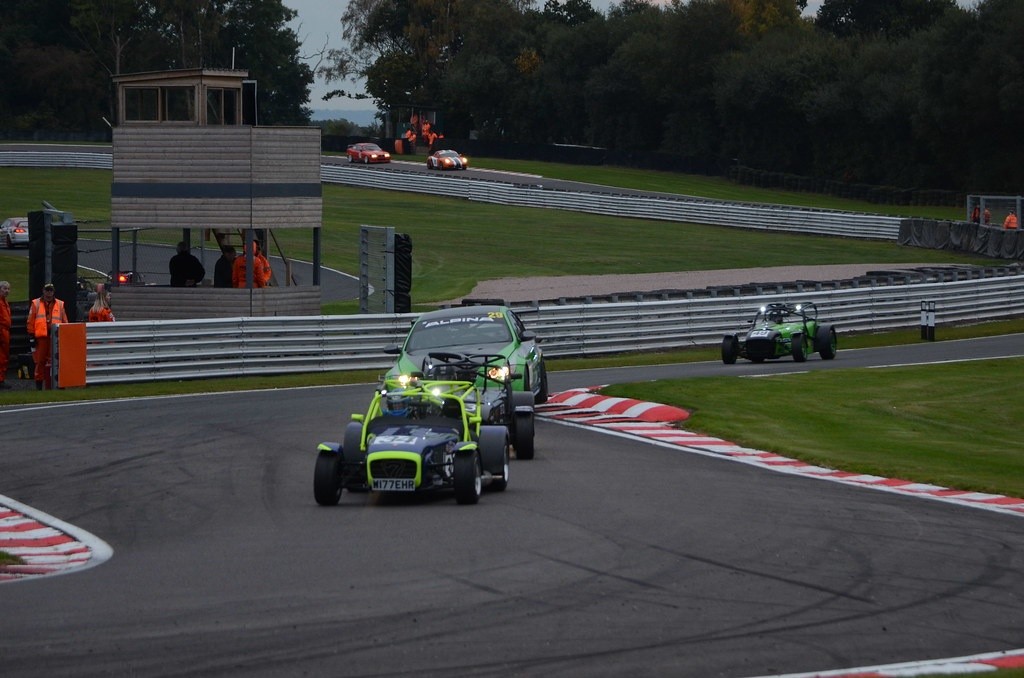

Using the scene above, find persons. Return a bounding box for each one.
[214,247,239,288]
[971,205,991,226]
[27,282,69,391]
[0,279,13,388]
[252,238,272,282]
[169,241,206,287]
[404,108,445,150]
[766,311,784,323]
[88,286,115,368]
[381,389,417,418]
[232,241,265,288]
[1002,209,1018,229]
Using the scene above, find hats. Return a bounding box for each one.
[43,283,56,291]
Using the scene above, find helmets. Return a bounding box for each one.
[442,397,462,407]
[387,388,410,405]
[434,363,454,376]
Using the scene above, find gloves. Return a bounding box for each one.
[28,335,38,348]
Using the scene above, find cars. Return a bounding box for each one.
[380,306,551,406]
[347,142,390,167]
[428,148,469,170]
[0,217,31,251]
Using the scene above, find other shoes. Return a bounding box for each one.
[36,382,44,390]
[1,381,12,390]
